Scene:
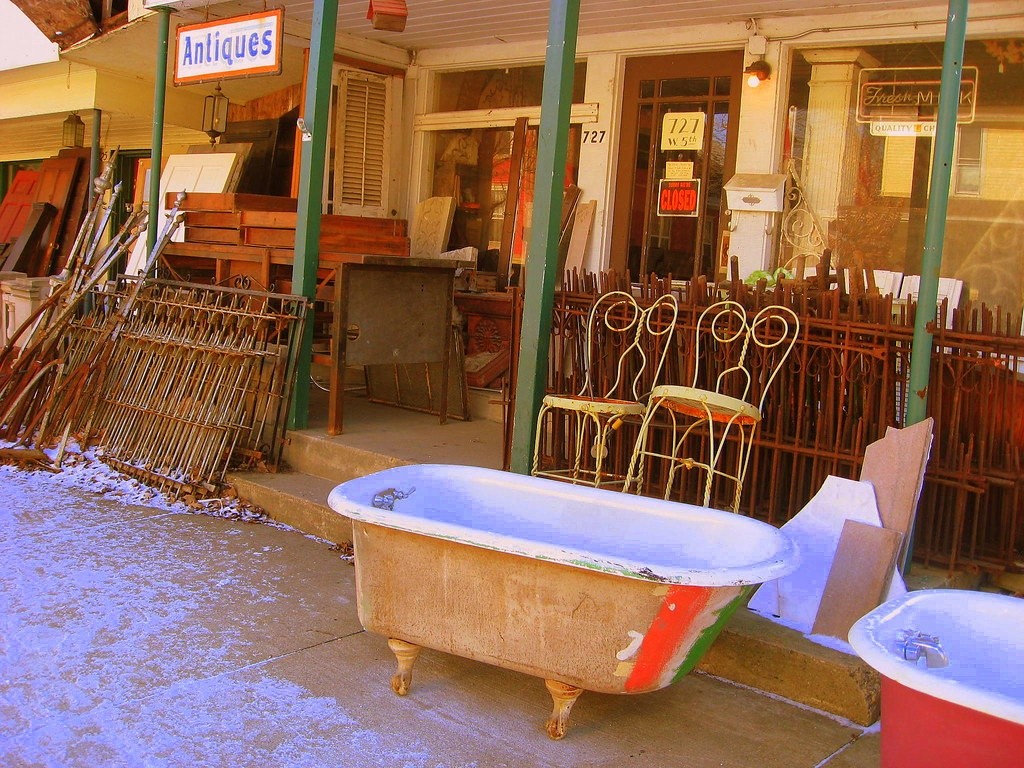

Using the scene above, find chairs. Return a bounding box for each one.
[530,289,800,516]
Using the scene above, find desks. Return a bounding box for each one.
[147,242,478,437]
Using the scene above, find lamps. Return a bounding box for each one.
[62,110,85,149]
[202,81,229,147]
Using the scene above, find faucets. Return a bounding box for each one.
[903,628,951,672]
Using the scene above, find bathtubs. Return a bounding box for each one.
[326,462,801,743]
[847,588,1023,768]
